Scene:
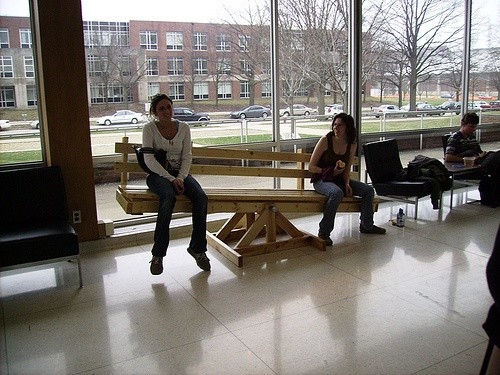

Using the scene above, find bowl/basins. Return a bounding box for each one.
[463,157,474,166]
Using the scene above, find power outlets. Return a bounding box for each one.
[73,210,82,223]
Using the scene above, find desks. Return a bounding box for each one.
[447,164,481,209]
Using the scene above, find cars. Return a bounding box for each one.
[436,99,500,115]
[96,109,143,125]
[230,105,271,118]
[324,103,343,120]
[478,94,492,99]
[400,102,424,111]
[371,104,407,118]
[416,104,445,117]
[30,120,40,129]
[280,104,312,117]
[0,119,11,131]
[440,94,454,99]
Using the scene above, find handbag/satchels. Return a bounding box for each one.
[310,133,335,167]
[133,144,172,175]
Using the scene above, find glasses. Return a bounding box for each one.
[464,124,477,128]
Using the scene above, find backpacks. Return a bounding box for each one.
[479,170,500,208]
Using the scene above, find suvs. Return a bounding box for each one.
[173,108,210,127]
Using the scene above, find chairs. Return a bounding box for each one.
[364,138,454,220]
[442,133,496,202]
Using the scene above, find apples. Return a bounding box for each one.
[337,160,346,168]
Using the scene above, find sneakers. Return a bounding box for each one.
[187,248,210,271]
[149,256,163,275]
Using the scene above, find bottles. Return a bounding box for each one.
[397,209,405,227]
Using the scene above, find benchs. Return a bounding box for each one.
[116,137,386,215]
[0,164,83,291]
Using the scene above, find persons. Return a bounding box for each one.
[309,113,386,245]
[445,113,500,208]
[141,93,211,275]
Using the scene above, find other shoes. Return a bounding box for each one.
[318,232,333,246]
[359,223,386,234]
